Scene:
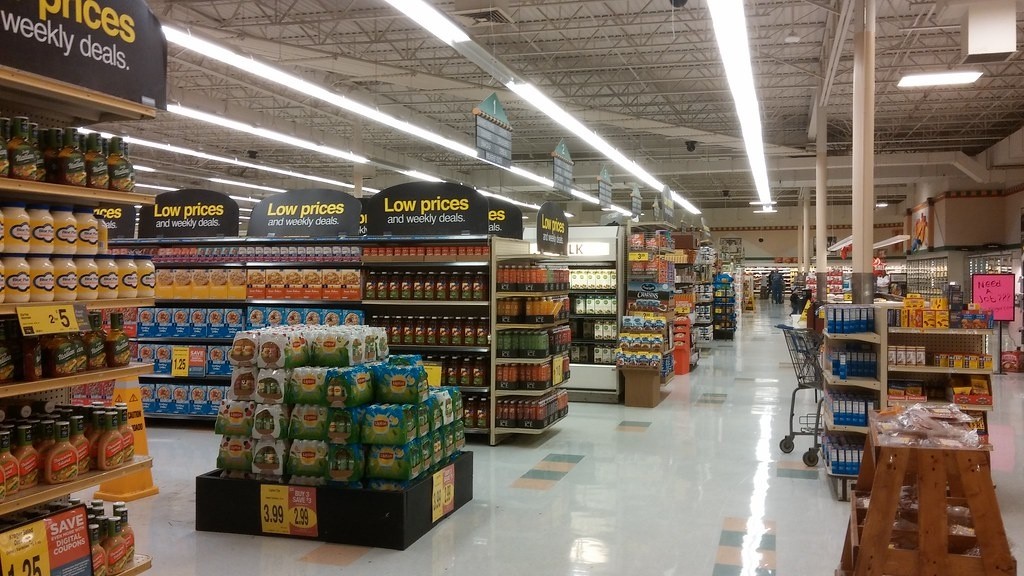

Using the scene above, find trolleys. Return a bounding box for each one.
[769,279,786,303]
[774,324,824,467]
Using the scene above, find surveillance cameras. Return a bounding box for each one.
[686,141,698,152]
[247,150,258,159]
[670,0,687,7]
[759,238,763,242]
[722,190,729,197]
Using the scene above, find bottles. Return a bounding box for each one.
[69,498,133,576]
[0,310,132,381]
[0,401,134,502]
[214,324,466,483]
[364,271,490,428]
[0,115,136,194]
[497,262,572,429]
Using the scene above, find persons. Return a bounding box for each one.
[757,269,783,304]
[789,288,800,316]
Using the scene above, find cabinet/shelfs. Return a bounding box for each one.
[521,221,678,405]
[0,67,161,576]
[821,302,995,501]
[669,234,851,366]
[835,410,1017,576]
[87,238,571,446]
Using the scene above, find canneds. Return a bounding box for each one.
[0,202,155,305]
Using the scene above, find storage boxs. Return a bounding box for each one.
[567,270,616,364]
[611,365,661,407]
[823,294,993,476]
[672,235,700,250]
[71,245,363,415]
